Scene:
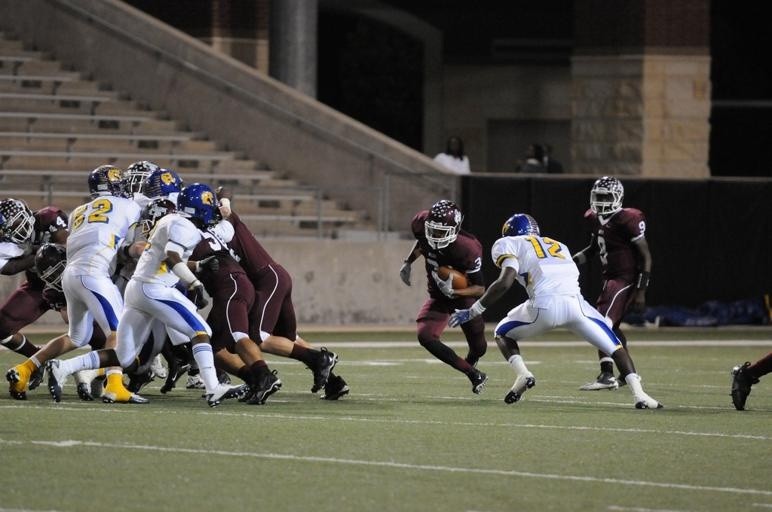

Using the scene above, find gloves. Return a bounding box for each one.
[431,271,454,299]
[188,279,208,310]
[448,308,478,329]
[399,261,411,287]
[196,255,219,273]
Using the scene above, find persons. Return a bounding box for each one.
[0,161,350,401]
[571,176,652,391]
[729,351,772,410]
[432,136,470,175]
[515,143,542,173]
[448,213,663,410]
[398,199,486,395]
[543,144,563,172]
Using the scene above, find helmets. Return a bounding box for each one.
[143,168,185,198]
[88,164,131,199]
[589,177,624,215]
[0,198,36,244]
[142,198,175,238]
[34,243,67,294]
[125,161,157,194]
[501,214,540,239]
[425,200,463,250]
[177,183,217,231]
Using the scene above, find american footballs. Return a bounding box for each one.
[439,266,467,289]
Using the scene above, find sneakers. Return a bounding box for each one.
[320,376,350,401]
[504,372,537,404]
[466,366,488,394]
[463,355,479,368]
[732,363,759,411]
[237,370,282,405]
[161,363,192,394]
[203,383,250,407]
[126,369,156,394]
[311,347,338,393]
[101,382,150,405]
[186,373,206,390]
[632,393,665,410]
[6,366,27,401]
[29,345,49,392]
[150,353,167,379]
[71,369,94,401]
[201,373,231,398]
[578,377,619,391]
[616,374,628,387]
[45,358,66,404]
